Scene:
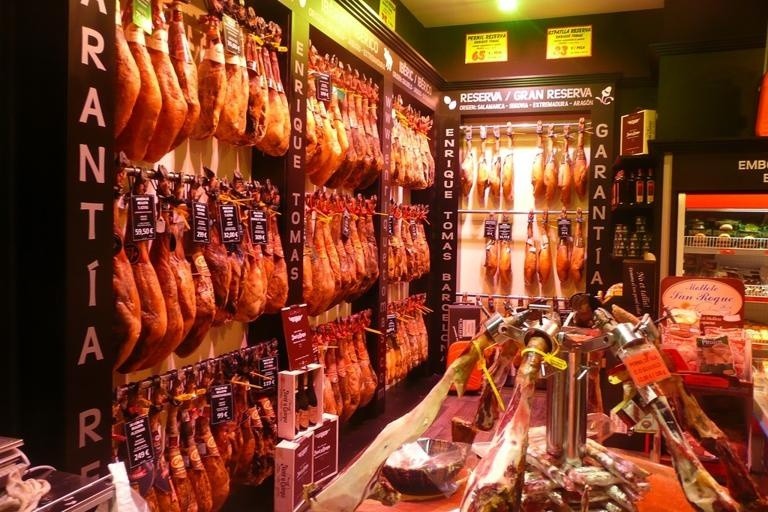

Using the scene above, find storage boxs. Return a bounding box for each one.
[274,303,340,512]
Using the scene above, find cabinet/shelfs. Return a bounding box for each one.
[608,153,656,263]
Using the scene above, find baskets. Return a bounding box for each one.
[381,438,467,493]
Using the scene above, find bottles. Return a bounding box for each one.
[622,165,656,206]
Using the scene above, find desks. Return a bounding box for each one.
[1,467,116,511]
